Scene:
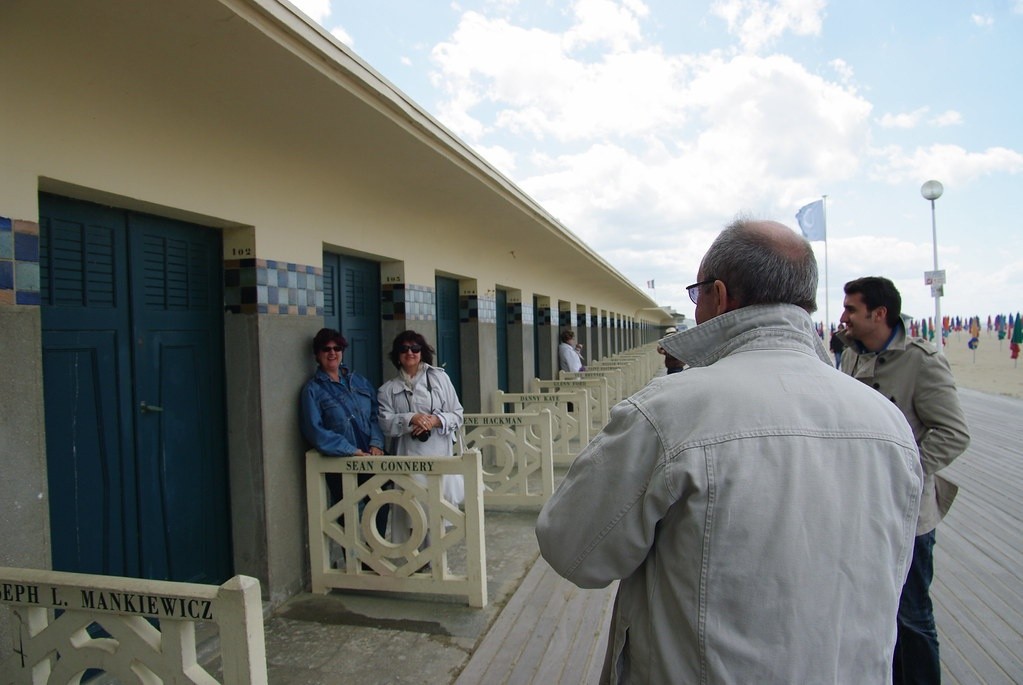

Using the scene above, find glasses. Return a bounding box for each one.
[686,279,735,305]
[321,345,344,352]
[399,345,422,353]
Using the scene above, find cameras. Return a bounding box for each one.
[411,428,431,442]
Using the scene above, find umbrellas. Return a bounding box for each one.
[995,312,1023,368]
[911,314,992,364]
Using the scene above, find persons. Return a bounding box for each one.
[378,331,465,570]
[559,331,583,412]
[831,324,845,369]
[301,328,389,571]
[537,220,925,685]
[658,328,686,373]
[836,277,969,685]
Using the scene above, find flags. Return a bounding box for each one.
[647,280,654,288]
[796,199,825,241]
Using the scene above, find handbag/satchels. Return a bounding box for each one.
[348,418,391,487]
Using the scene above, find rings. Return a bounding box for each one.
[423,422,426,424]
